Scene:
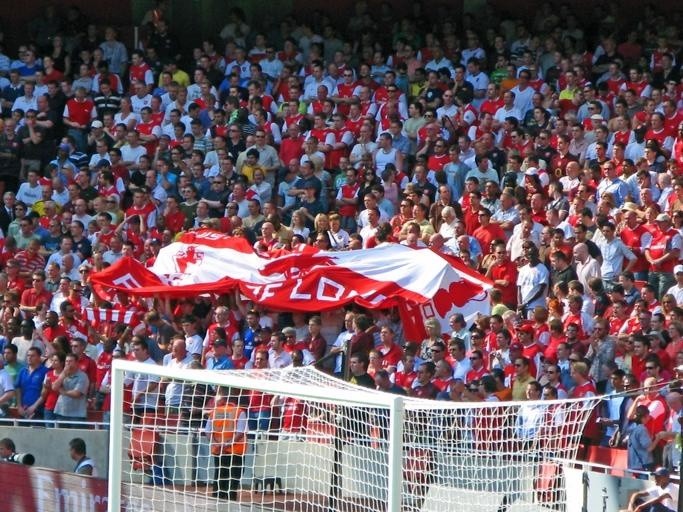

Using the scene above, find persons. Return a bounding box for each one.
[0,0,683,511]
